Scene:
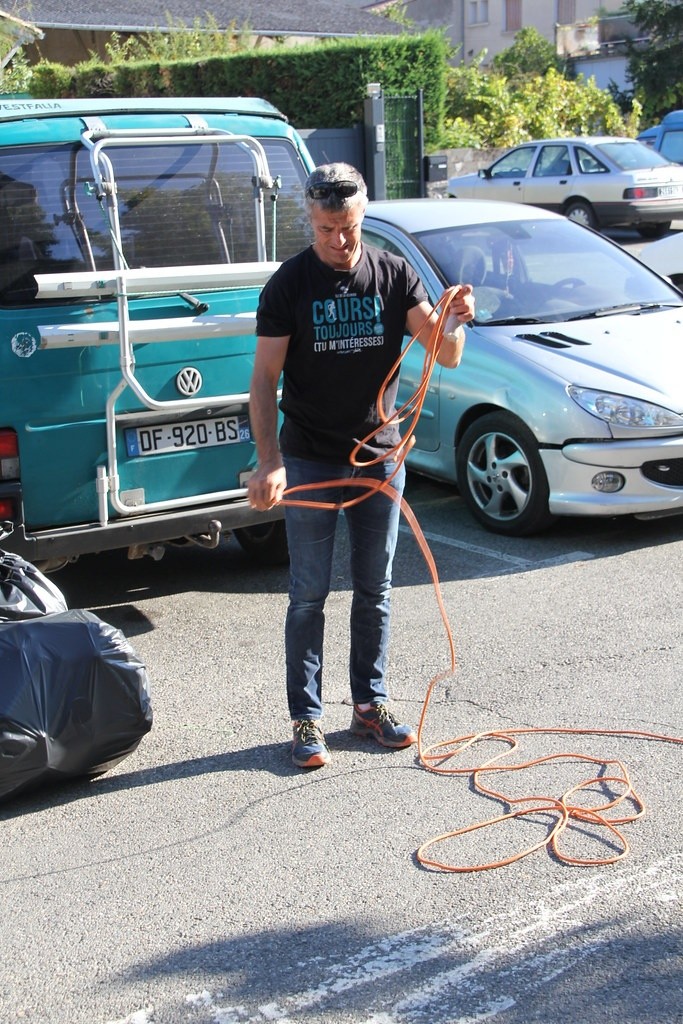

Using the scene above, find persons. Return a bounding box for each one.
[248,160,476,771]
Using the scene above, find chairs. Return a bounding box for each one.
[448,246,528,322]
[542,160,571,176]
[584,159,592,172]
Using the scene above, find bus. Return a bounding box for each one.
[0,91,318,574]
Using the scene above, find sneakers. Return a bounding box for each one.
[290,719,331,767]
[350,703,417,747]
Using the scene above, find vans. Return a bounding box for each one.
[638,107,683,163]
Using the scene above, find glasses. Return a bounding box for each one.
[307,180,357,201]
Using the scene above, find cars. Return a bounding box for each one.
[360,197,683,536]
[447,136,682,239]
